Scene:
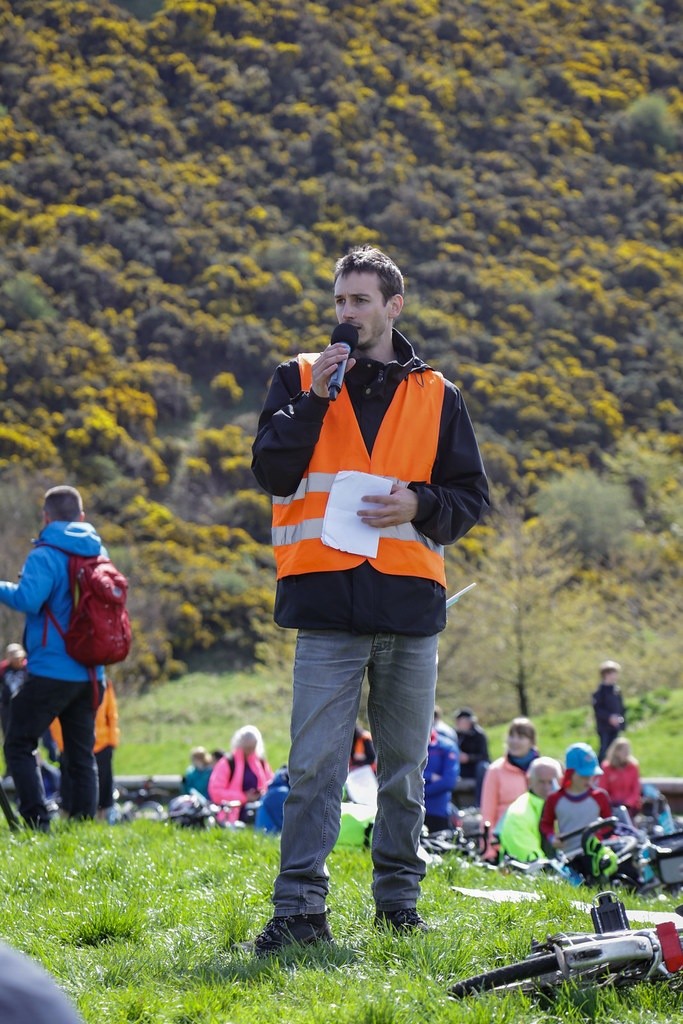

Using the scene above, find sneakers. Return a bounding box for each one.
[231,913,332,956]
[374,910,428,936]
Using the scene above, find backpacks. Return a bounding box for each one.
[37,543,131,665]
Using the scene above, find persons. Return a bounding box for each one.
[595,737,643,815]
[480,717,540,859]
[208,726,275,828]
[230,248,491,959]
[0,485,110,833]
[0,643,58,762]
[255,765,291,836]
[349,723,377,776]
[500,756,562,864]
[50,674,120,820]
[592,661,626,763]
[455,708,491,808]
[423,708,461,834]
[181,747,226,801]
[538,743,618,852]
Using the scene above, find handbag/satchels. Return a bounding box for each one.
[256,766,288,834]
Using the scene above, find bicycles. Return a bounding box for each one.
[446,890,683,1012]
[111,776,242,831]
[441,817,683,897]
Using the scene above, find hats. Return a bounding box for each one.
[565,743,604,776]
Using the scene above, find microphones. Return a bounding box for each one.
[327,323,359,401]
